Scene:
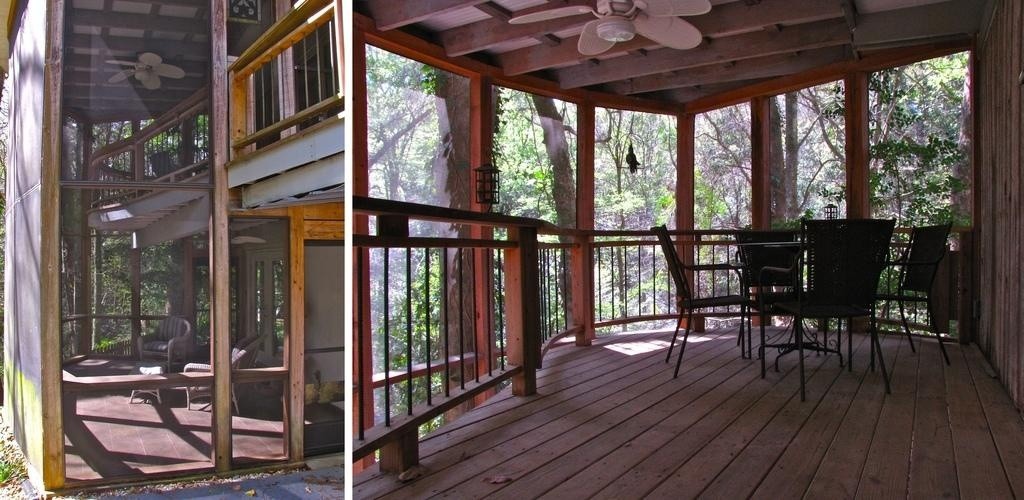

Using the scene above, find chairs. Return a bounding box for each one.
[871,221,954,374]
[732,229,801,360]
[651,224,761,379]
[136,317,191,373]
[150,151,176,178]
[182,332,268,416]
[755,217,897,402]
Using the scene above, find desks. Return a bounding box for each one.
[736,242,846,372]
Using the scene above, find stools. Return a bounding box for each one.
[128,359,168,405]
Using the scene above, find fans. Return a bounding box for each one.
[507,0,712,56]
[105,52,185,90]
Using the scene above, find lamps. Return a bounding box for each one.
[596,20,635,42]
[133,67,151,82]
